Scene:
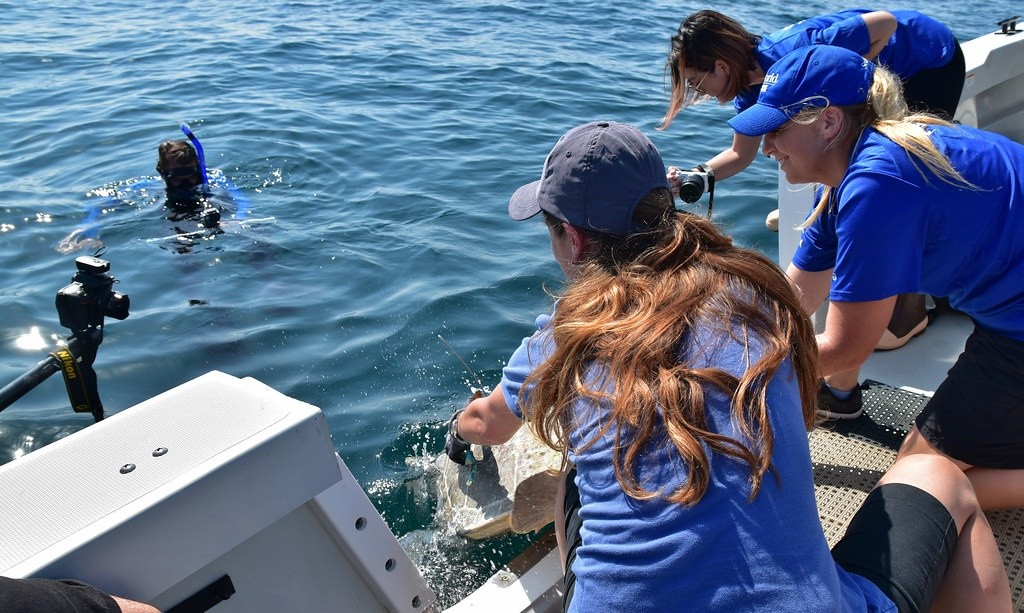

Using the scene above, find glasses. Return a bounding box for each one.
[687,65,713,93]
[165,165,199,182]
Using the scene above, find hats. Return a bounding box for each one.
[508,121,674,237]
[726,44,876,137]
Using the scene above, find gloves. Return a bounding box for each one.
[444,409,483,466]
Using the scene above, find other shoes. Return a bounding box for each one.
[813,378,862,418]
[874,315,928,350]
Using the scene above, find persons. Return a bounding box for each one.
[727,44,1024,511]
[157,139,200,194]
[448,8,1024,613]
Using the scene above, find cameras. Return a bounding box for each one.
[674,171,711,204]
[55,256,129,330]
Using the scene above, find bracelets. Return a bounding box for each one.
[450,415,461,437]
